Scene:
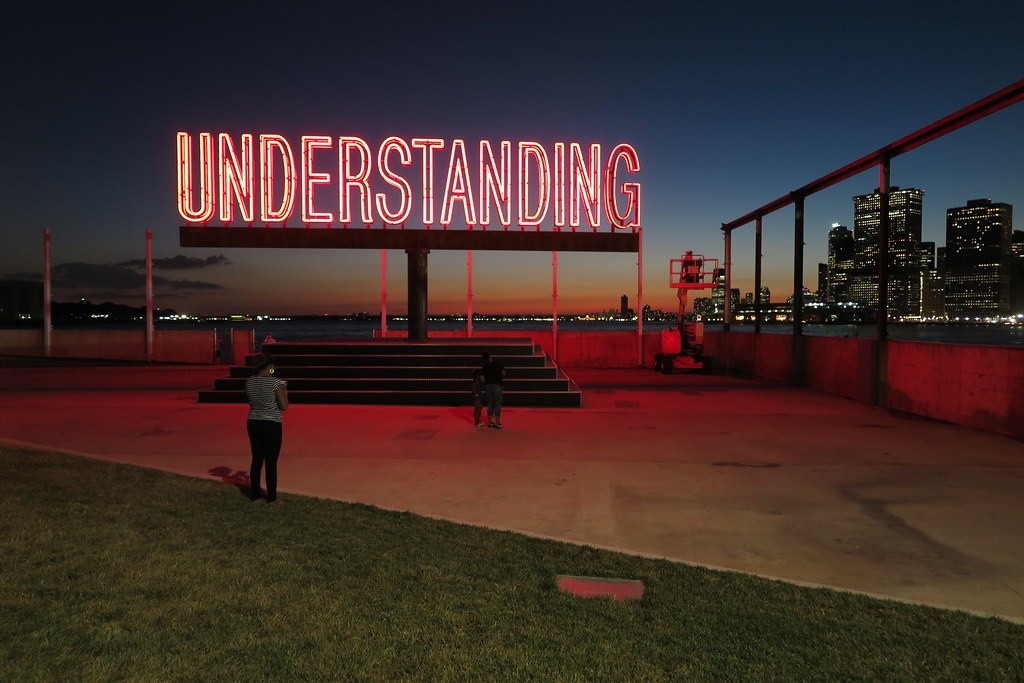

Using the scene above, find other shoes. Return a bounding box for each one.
[476,423,485,427]
[488,422,503,429]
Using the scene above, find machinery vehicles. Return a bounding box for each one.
[649,250,721,374]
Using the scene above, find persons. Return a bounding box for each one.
[246,354,291,505]
[471,351,506,429]
[262,330,279,343]
[680,251,703,284]
